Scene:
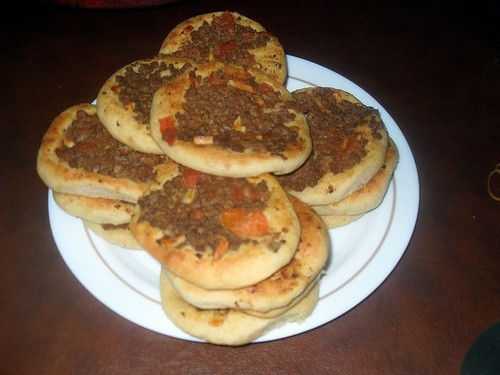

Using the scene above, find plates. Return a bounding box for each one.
[47,55,419,344]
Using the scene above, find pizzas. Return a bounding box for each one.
[268,87,399,230]
[127,165,331,347]
[148,61,312,176]
[159,10,290,83]
[35,102,181,251]
[95,55,202,157]
